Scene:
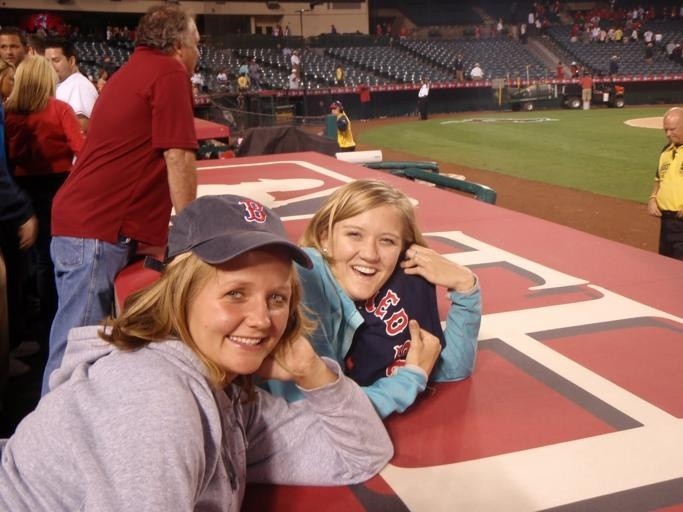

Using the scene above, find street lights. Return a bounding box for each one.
[266,0,324,116]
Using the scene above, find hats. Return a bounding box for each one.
[144,194,313,273]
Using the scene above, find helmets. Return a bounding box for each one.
[330,99,342,109]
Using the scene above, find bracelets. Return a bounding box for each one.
[648,192,656,200]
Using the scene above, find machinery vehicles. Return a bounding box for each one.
[509,80,626,113]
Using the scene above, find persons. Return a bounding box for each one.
[40,2,200,403]
[0,194,392,512]
[556,55,620,112]
[282,45,302,89]
[496,0,682,64]
[1,26,31,65]
[330,101,355,151]
[474,25,480,39]
[417,79,429,120]
[97,70,107,92]
[328,48,335,57]
[356,81,371,121]
[647,107,683,262]
[470,62,484,80]
[336,64,346,87]
[253,178,482,422]
[44,40,99,132]
[1,55,85,376]
[453,55,468,81]
[376,22,409,40]
[106,26,131,42]
[278,26,291,36]
[191,55,264,93]
